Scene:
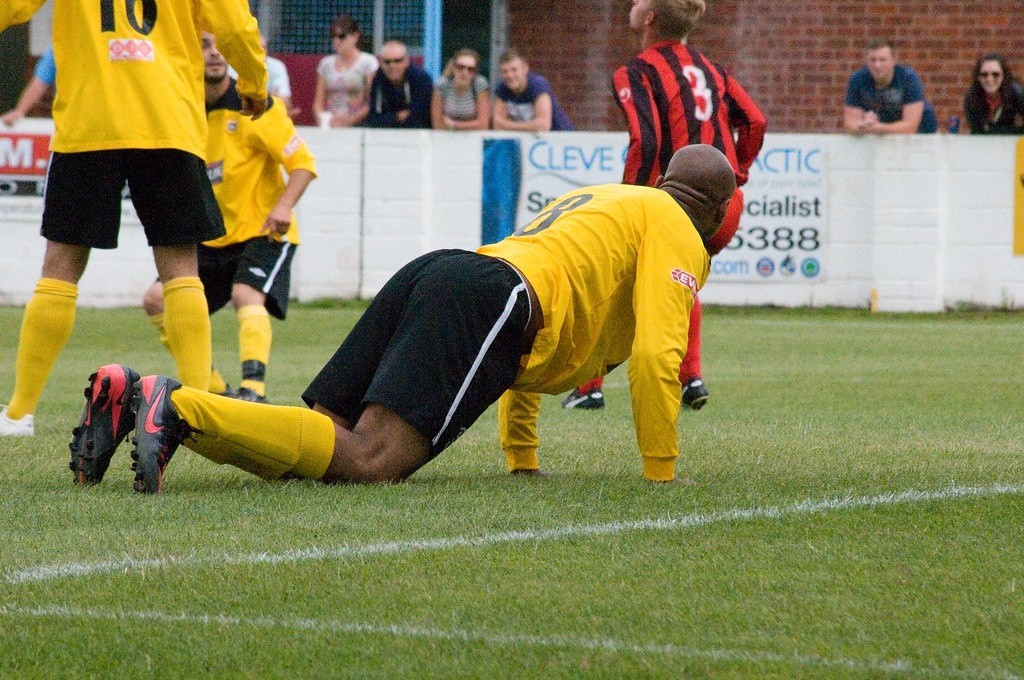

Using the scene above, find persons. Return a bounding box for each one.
[432,48,490,130]
[312,14,380,127]
[2,45,56,126]
[561,0,767,410]
[0,0,269,436]
[143,31,319,404]
[69,143,736,495]
[493,48,574,132]
[843,38,938,135]
[365,42,434,129]
[227,35,291,109]
[964,53,1024,135]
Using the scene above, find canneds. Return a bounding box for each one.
[949,116,959,133]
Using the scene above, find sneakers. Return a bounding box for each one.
[683,376,710,410]
[70,364,140,486]
[0,404,35,437]
[562,385,606,407]
[131,375,204,495]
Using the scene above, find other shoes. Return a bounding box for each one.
[219,384,235,398]
[233,387,268,403]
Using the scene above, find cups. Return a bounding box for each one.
[319,111,333,128]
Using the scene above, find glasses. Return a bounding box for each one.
[978,72,1001,77]
[381,58,405,65]
[454,63,478,73]
[330,32,347,39]
